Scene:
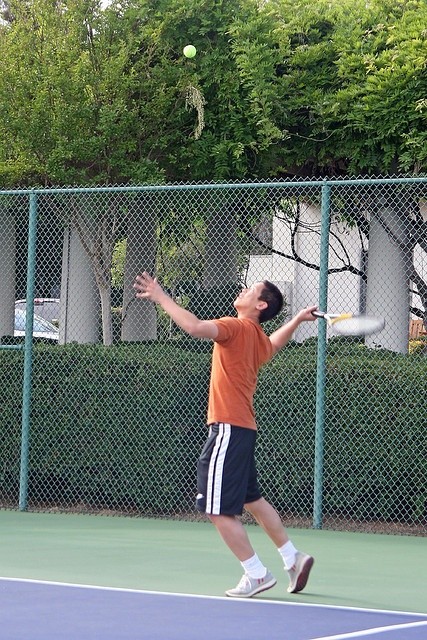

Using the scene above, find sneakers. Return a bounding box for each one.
[284,551,314,593]
[225,567,277,597]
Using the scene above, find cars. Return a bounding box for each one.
[13,308,60,341]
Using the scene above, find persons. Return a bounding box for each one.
[132,270,320,598]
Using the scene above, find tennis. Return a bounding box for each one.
[183,45,196,58]
